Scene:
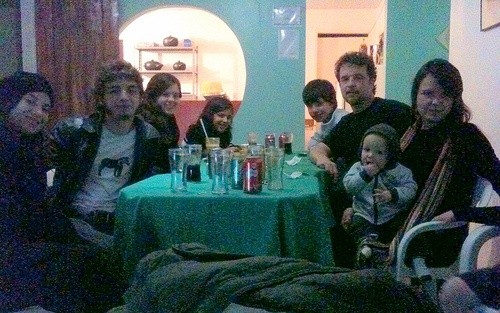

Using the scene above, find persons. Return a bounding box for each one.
[137,74,182,180]
[343,124,418,264]
[43,59,162,303]
[310,52,414,239]
[181,97,240,153]
[340,58,500,268]
[0,69,87,312]
[302,77,351,152]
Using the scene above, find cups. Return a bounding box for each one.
[168,148,188,193]
[184,39,192,47]
[182,145,202,183]
[264,148,284,190]
[282,132,293,154]
[209,148,230,194]
[249,134,256,145]
[206,137,220,154]
[249,145,265,184]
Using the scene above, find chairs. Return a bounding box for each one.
[396,176,500,282]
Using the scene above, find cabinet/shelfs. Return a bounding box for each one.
[137,44,198,100]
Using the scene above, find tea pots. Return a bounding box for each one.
[145,60,163,70]
[162,36,178,46]
[173,60,186,70]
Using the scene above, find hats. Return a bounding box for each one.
[0,71,55,110]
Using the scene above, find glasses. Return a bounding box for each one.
[20,97,53,113]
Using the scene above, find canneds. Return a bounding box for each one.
[208,147,223,179]
[231,155,245,189]
[242,156,262,192]
[279,134,288,148]
[265,134,275,148]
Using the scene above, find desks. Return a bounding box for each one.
[113,151,333,271]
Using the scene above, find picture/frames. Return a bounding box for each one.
[480,0,500,32]
